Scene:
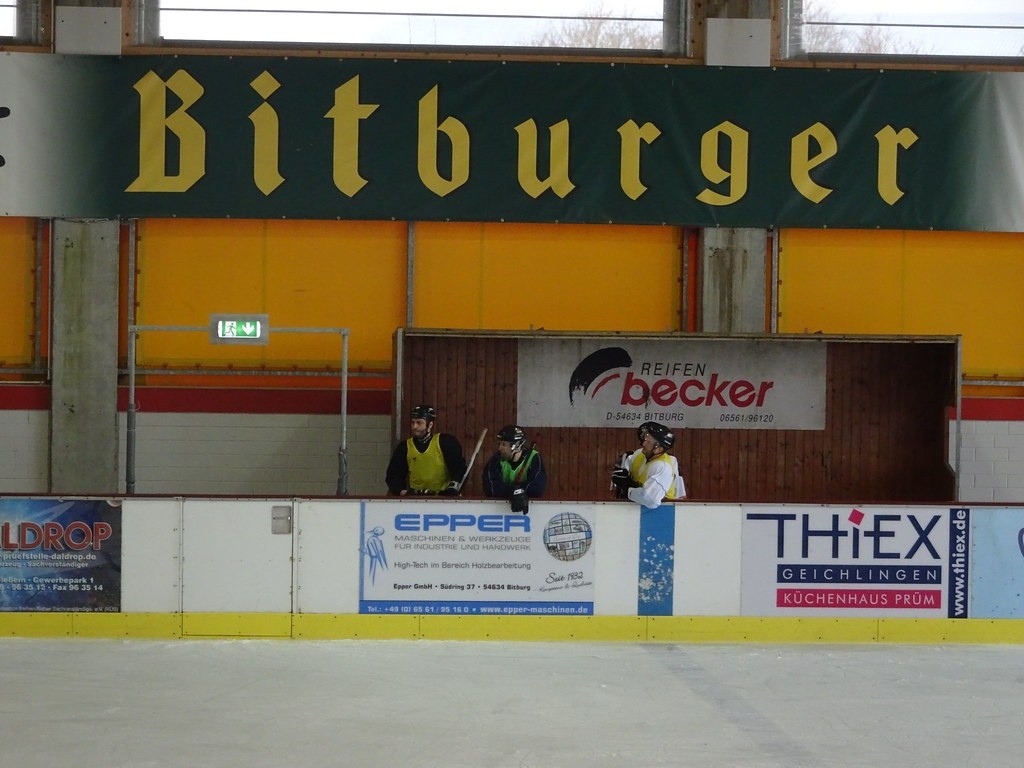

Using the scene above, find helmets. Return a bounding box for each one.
[496,424,526,455]
[410,405,437,419]
[646,422,676,451]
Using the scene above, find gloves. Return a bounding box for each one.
[444,488,458,496]
[511,489,529,515]
[404,490,414,496]
[612,466,639,502]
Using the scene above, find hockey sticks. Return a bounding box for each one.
[457,428,488,493]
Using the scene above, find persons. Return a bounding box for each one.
[482,425,546,498]
[611,422,686,509]
[384,404,469,497]
